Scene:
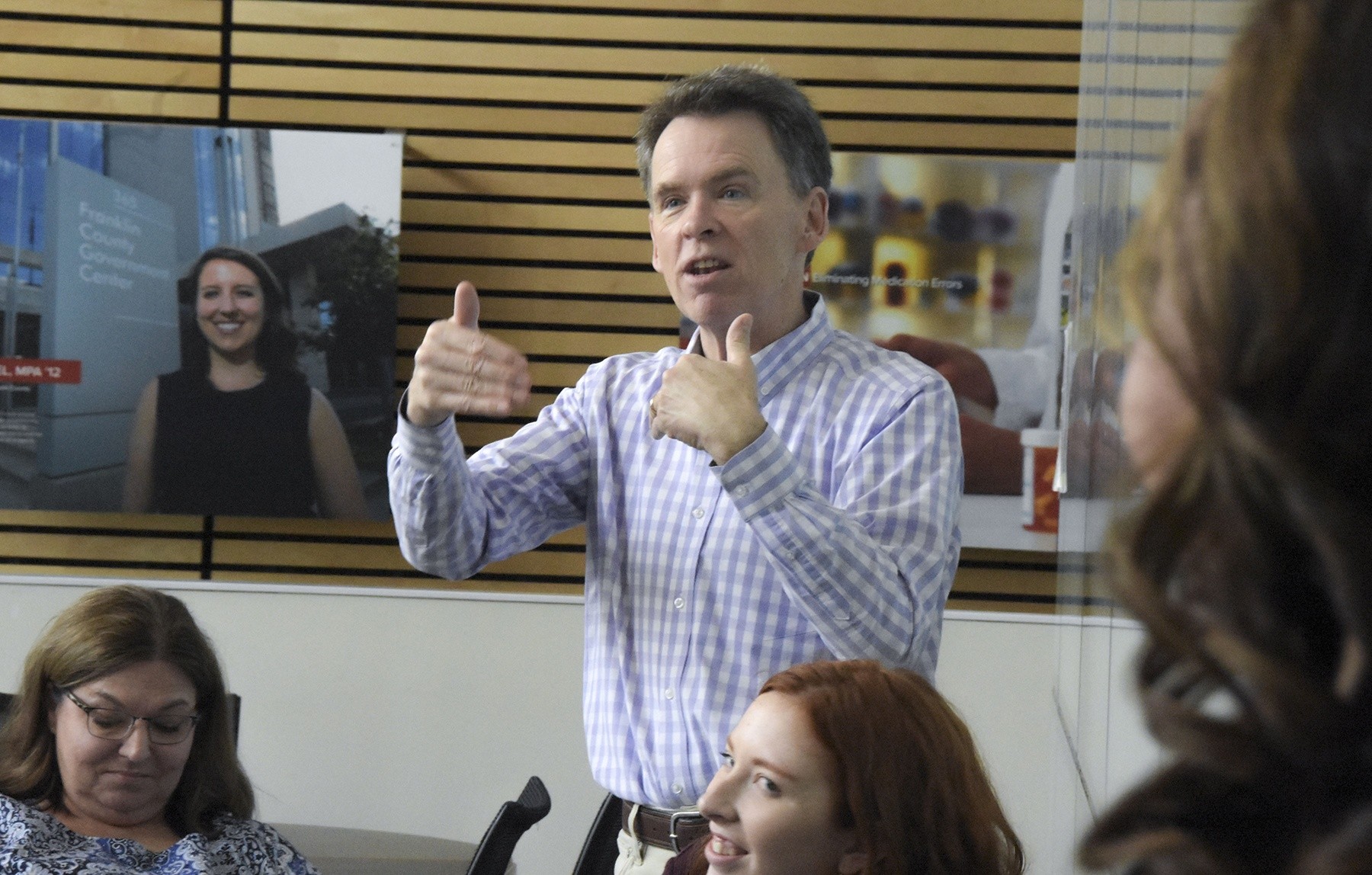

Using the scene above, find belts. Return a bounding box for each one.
[622,800,710,854]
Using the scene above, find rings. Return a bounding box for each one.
[649,398,657,416]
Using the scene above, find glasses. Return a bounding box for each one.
[62,688,202,745]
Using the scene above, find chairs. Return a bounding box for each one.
[467,777,551,875]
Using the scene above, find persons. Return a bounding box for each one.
[122,246,372,528]
[383,63,965,875]
[867,162,1164,494]
[0,584,324,875]
[661,657,1029,875]
[1061,0,1372,875]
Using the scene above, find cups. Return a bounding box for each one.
[1018,426,1062,531]
